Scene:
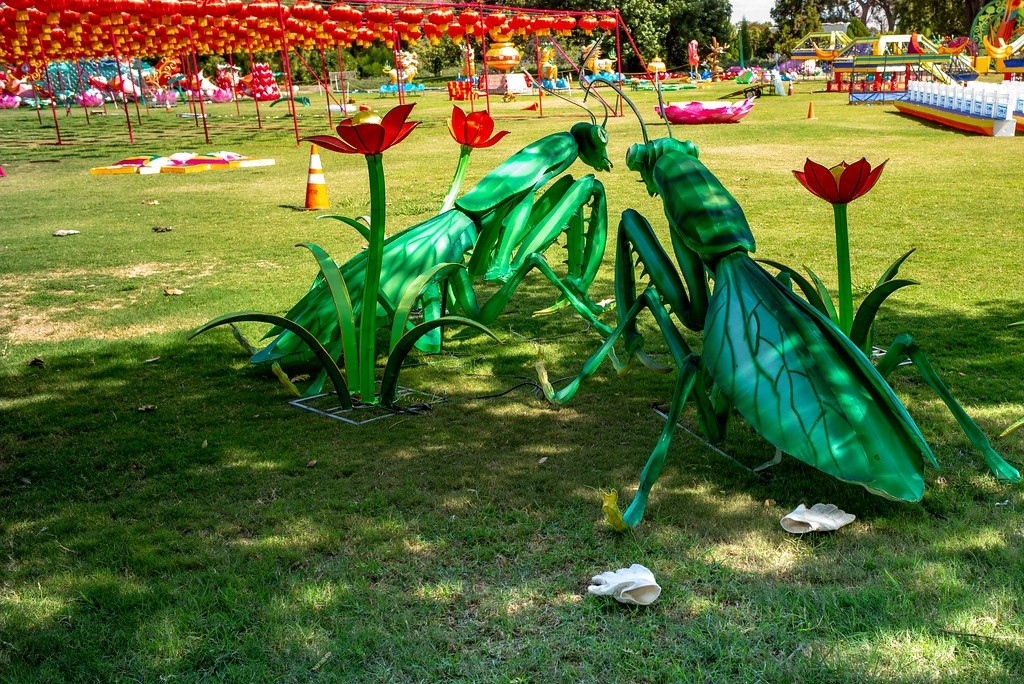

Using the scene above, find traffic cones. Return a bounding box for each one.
[359,104,371,111]
[787,80,794,96]
[521,102,539,111]
[807,101,816,119]
[296,144,333,211]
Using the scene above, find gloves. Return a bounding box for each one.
[779,502,856,534]
[588,563,663,606]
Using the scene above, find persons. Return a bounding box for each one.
[749,72,759,83]
